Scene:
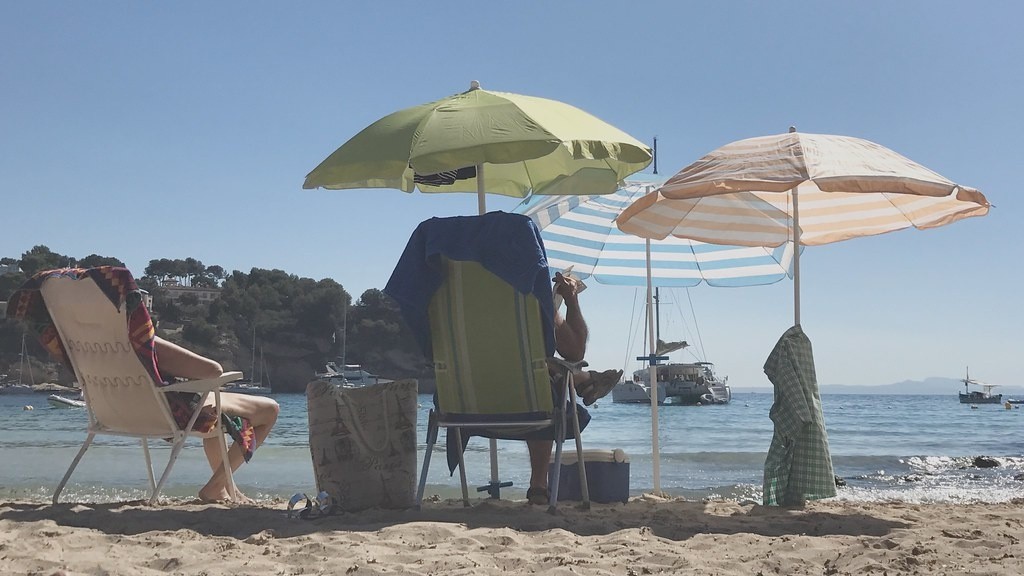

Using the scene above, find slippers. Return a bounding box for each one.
[313,491,345,516]
[283,493,316,520]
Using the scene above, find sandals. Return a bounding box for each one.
[576,368,624,406]
[526,487,551,505]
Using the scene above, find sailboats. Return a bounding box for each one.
[314,288,394,389]
[610,135,732,407]
[177,326,272,396]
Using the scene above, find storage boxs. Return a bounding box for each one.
[543,449,630,504]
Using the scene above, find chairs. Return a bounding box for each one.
[4,266,245,504]
[383,210,593,514]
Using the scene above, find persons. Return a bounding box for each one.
[487,210,623,504]
[78,255,279,503]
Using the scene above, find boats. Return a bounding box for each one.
[1008,399,1024,405]
[958,365,1002,404]
[44,387,86,408]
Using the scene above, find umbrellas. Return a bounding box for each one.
[303,79,653,214]
[617,125,988,326]
[522,174,803,490]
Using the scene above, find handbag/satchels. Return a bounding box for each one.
[307,378,420,516]
